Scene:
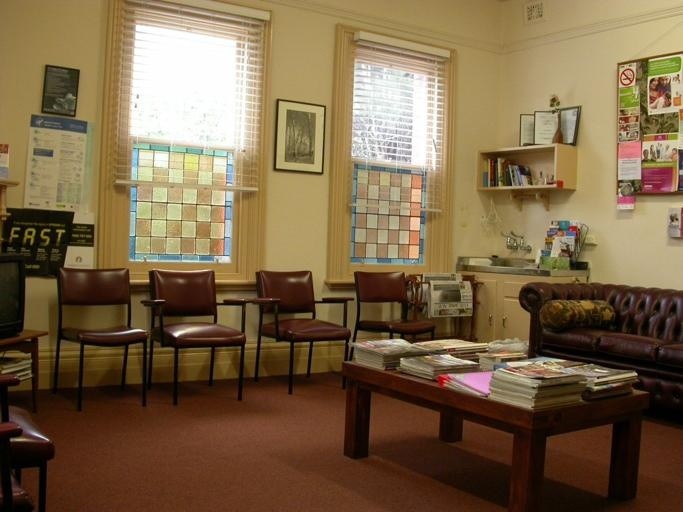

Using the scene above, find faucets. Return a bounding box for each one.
[500,231,532,252]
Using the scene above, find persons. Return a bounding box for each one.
[650,76,671,108]
[641,141,678,160]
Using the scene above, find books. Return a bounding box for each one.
[0,350,32,380]
[350,337,640,411]
[483,157,531,185]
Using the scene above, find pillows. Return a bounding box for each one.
[539,297,619,332]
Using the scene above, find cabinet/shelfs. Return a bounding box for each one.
[475,146,578,192]
[459,272,585,343]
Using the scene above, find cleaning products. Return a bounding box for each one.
[535,249,542,264]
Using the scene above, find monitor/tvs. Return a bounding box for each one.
[0,253,25,337]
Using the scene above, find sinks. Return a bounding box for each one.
[456,256,530,274]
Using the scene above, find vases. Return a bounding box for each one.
[553,114,563,143]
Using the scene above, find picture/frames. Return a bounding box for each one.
[558,105,581,145]
[274,99,328,174]
[41,64,80,116]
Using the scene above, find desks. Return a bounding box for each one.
[0,330,49,422]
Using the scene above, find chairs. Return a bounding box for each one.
[248,267,355,394]
[52,267,152,410]
[348,268,435,378]
[1,374,56,512]
[140,267,254,410]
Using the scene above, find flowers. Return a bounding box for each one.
[549,94,561,113]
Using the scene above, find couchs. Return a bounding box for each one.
[519,282,683,428]
